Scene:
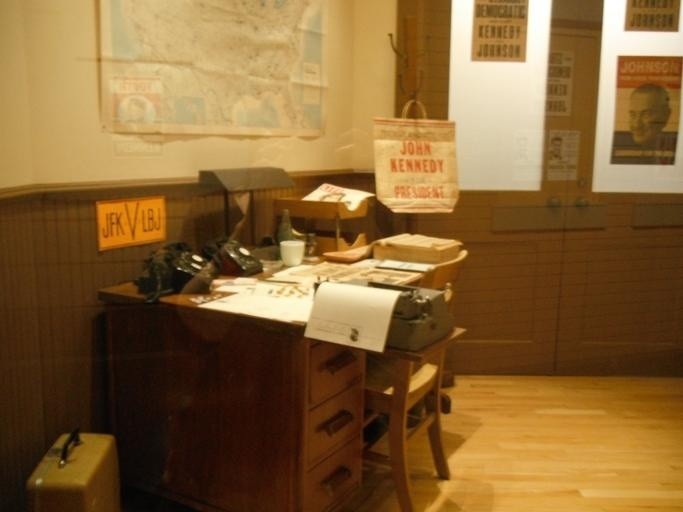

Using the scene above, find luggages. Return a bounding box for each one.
[22,428,126,512]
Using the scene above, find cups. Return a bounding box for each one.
[280,240,305,266]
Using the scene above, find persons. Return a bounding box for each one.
[611,83,672,165]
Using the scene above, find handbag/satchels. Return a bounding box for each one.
[373,99,461,214]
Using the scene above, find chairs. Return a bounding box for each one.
[361,248,472,511]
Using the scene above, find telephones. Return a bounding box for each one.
[141,237,263,293]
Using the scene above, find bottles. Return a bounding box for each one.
[276,208,293,260]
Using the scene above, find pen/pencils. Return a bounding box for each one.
[368,282,416,290]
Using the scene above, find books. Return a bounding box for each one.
[197,233,464,353]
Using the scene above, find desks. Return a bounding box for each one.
[97,255,456,512]
